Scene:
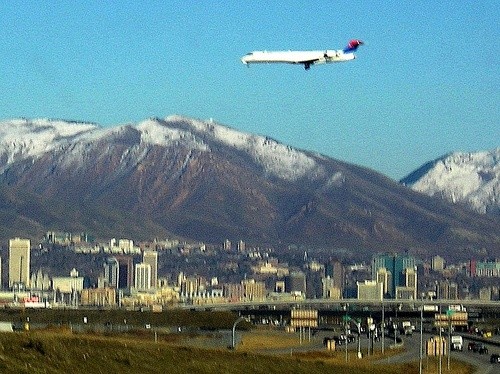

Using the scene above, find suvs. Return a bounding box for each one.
[335,334,351,345]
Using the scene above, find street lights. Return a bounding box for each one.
[232,317,250,348]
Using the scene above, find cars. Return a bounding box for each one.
[490,354,500,363]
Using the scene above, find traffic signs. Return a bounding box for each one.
[343,315,350,320]
[340,302,349,361]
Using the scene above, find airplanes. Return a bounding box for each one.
[240,39,363,69]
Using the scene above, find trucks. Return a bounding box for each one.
[400,321,413,335]
[451,336,463,351]
[468,342,484,352]
